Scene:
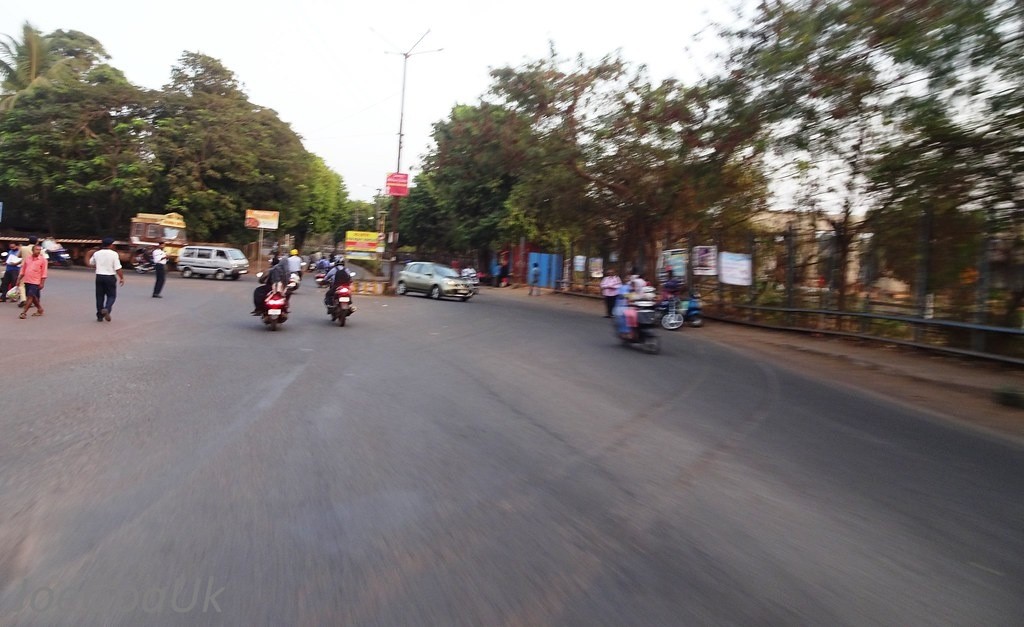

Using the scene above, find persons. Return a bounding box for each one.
[0,236,63,318]
[528,263,541,296]
[602,266,674,342]
[251,248,354,316]
[152,242,169,299]
[90,238,125,322]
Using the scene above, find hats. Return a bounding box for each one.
[102,237,115,245]
[271,258,280,265]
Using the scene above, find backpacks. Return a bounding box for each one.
[334,266,350,285]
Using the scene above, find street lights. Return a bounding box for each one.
[384,28,443,285]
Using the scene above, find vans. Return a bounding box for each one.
[175,246,249,280]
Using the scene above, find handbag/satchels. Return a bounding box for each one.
[6,286,21,302]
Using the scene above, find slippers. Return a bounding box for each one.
[31,310,45,316]
[19,313,27,319]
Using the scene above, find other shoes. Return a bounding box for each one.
[97,316,103,322]
[250,307,264,316]
[153,293,162,298]
[101,309,111,322]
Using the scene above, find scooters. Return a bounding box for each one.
[132,252,169,274]
[40,239,72,267]
[255,270,289,329]
[279,257,307,291]
[462,270,480,295]
[610,296,663,353]
[315,262,334,288]
[656,293,684,330]
[322,272,357,326]
[678,289,704,328]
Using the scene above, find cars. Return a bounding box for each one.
[395,262,474,301]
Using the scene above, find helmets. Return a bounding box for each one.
[290,249,299,255]
[334,254,345,264]
